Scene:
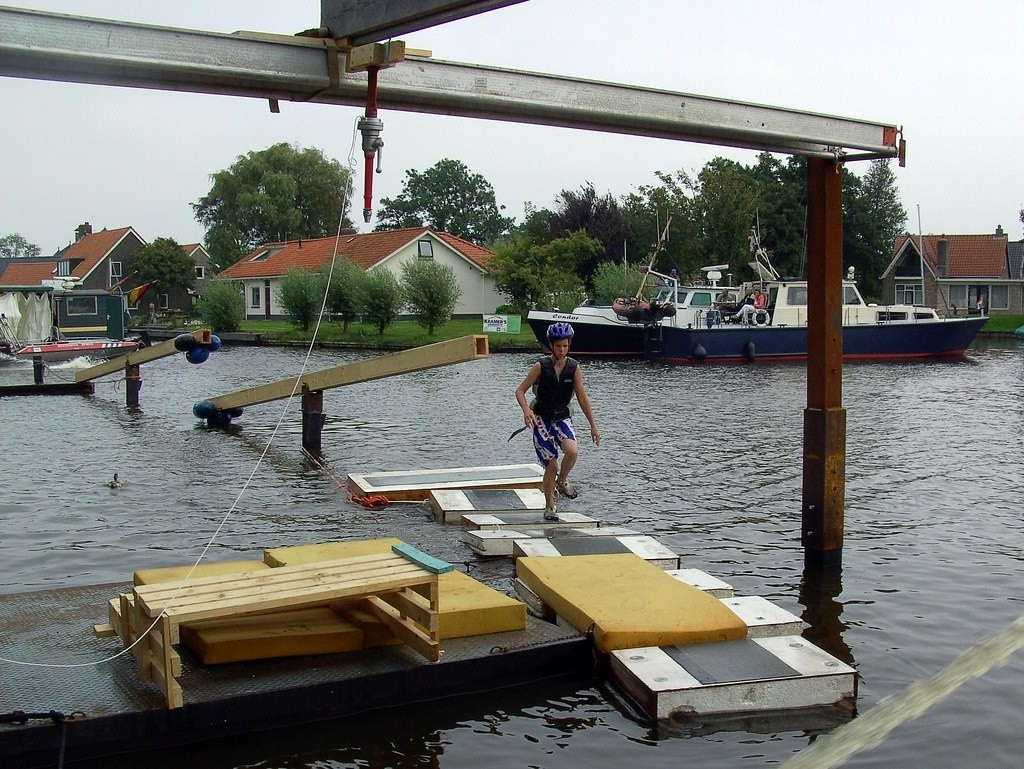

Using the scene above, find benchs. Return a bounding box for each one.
[92,552,440,711]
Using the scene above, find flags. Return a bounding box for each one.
[129,282,149,306]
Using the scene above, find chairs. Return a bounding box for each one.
[718,294,738,325]
[742,293,769,324]
[695,309,722,330]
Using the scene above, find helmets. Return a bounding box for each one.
[547,322,575,339]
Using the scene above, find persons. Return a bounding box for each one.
[976,297,983,310]
[712,286,765,325]
[515,323,599,520]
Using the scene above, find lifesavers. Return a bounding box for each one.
[751,309,771,327]
[611,297,651,317]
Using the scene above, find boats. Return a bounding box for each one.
[612,298,676,322]
[0,270,158,363]
[527,206,990,364]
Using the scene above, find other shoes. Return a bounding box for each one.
[731,315,738,320]
[543,508,559,520]
[557,479,578,499]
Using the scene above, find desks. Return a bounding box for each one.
[710,301,745,325]
[162,309,190,326]
